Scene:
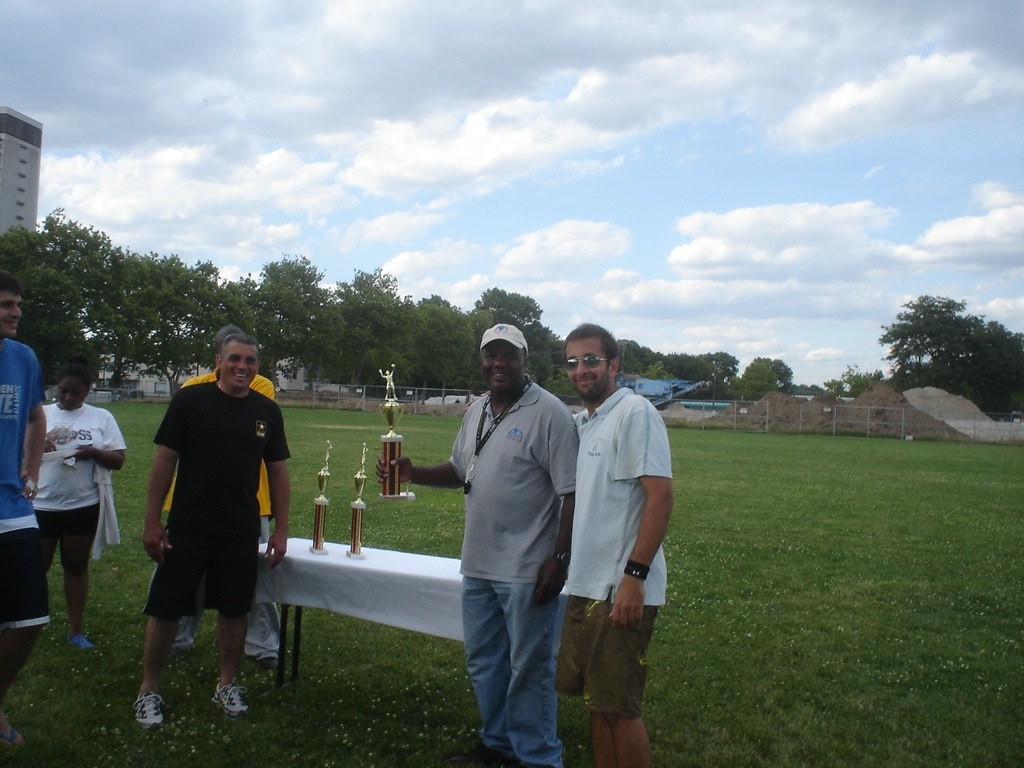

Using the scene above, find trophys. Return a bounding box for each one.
[313,440,333,549]
[350,443,368,553]
[378,363,404,496]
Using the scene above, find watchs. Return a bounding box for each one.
[552,551,571,565]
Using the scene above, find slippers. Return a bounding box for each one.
[0,727,24,746]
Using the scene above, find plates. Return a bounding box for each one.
[42,450,79,459]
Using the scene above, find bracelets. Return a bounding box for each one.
[624,560,650,580]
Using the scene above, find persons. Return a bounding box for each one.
[376,323,580,768]
[1,273,50,748]
[133,325,291,730]
[20,354,128,648]
[552,323,674,768]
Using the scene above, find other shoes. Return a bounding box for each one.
[434,744,520,767]
[253,654,278,669]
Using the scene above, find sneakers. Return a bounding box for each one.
[212,683,249,717]
[133,690,165,731]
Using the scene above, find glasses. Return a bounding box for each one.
[564,357,609,371]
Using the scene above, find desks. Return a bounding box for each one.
[255,537,568,689]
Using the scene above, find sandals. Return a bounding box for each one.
[67,632,95,650]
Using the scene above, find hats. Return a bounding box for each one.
[480,323,529,358]
